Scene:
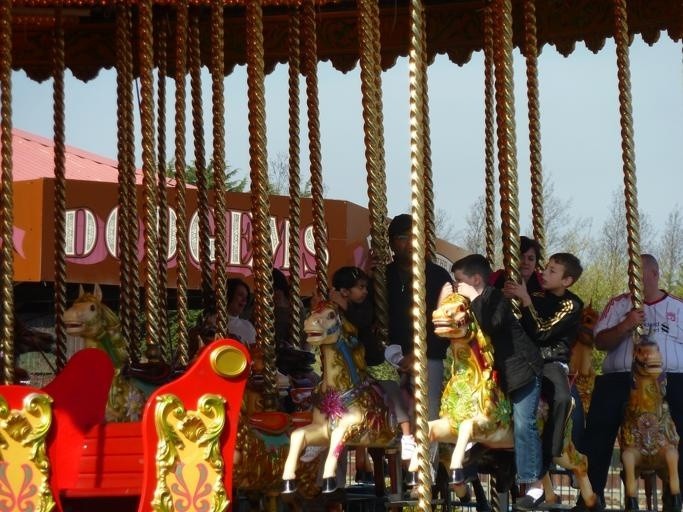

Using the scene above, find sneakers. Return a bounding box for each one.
[515,490,545,512]
[400,435,417,461]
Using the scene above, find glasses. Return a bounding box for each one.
[396,234,412,240]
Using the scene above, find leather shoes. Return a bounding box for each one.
[569,490,606,512]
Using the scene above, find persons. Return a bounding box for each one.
[574,255,681,510]
[356,214,454,503]
[451,237,584,510]
[204,268,368,411]
